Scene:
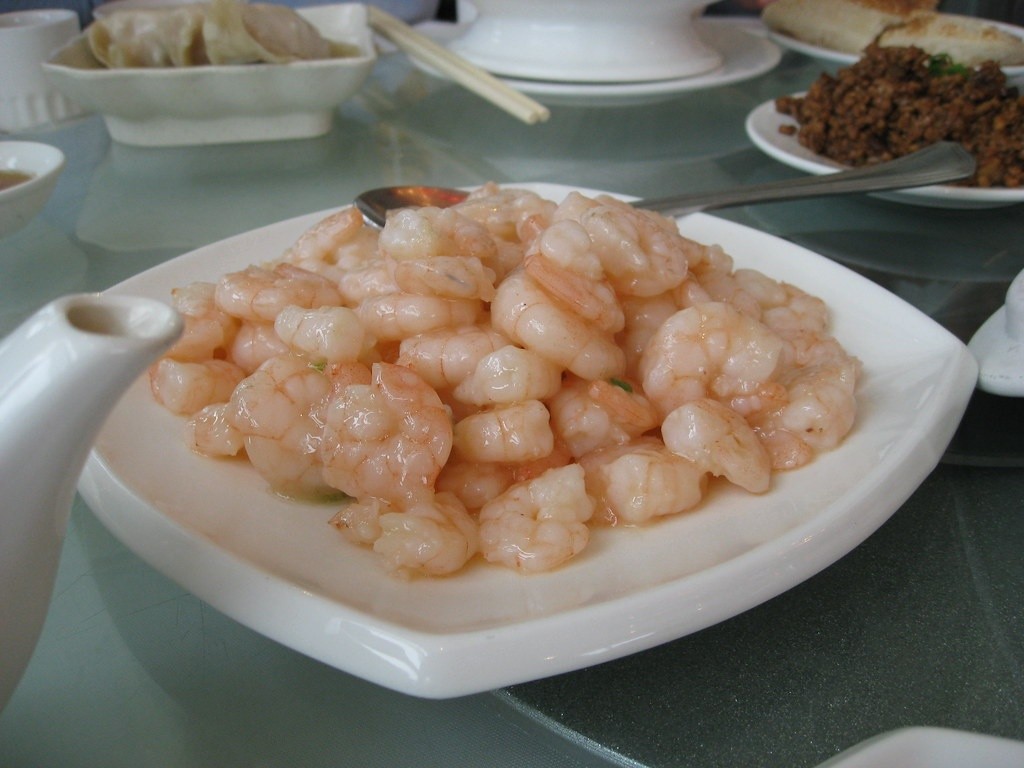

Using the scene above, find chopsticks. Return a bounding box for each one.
[367,5,550,125]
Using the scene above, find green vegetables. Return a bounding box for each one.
[928,53,969,81]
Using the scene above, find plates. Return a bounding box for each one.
[744,89,1024,209]
[404,16,783,109]
[767,12,1024,88]
[76,180,980,701]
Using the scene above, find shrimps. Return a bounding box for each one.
[148,180,866,579]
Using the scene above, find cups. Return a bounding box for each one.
[0,8,89,135]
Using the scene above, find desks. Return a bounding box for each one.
[0,13,1024,768]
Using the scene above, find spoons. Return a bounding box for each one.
[353,137,977,240]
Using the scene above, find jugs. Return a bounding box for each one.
[0,290,187,712]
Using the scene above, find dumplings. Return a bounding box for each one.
[88,0,330,68]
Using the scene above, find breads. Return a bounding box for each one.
[762,0,1024,66]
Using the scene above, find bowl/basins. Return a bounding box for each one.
[0,140,66,239]
[40,2,379,146]
[448,0,723,84]
